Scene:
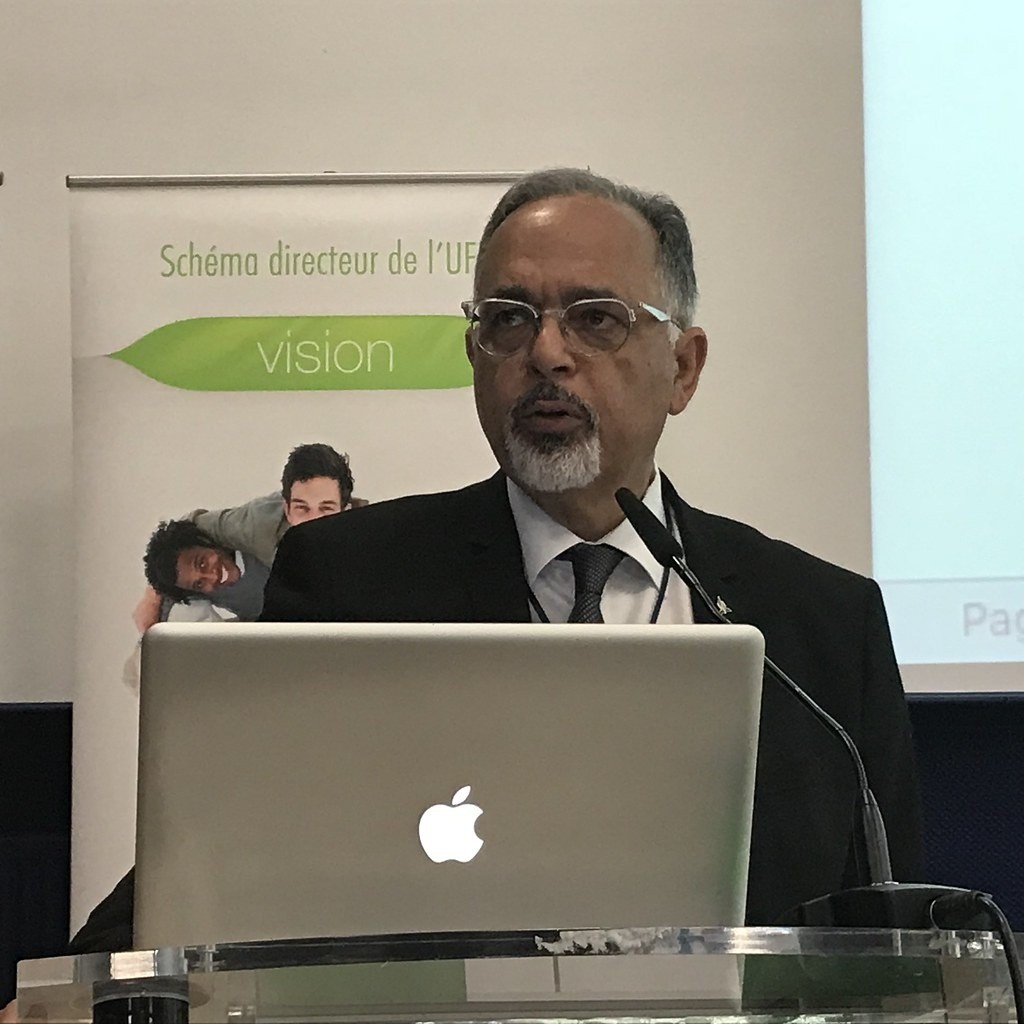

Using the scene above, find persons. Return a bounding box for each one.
[1,171,931,1018]
[129,444,373,635]
[145,522,276,632]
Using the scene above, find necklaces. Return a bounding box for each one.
[522,487,673,627]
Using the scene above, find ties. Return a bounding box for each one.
[564,544,623,624]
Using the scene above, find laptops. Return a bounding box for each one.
[132,620,764,1008]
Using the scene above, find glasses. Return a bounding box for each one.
[459,298,680,356]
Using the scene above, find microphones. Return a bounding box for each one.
[615,486,995,930]
